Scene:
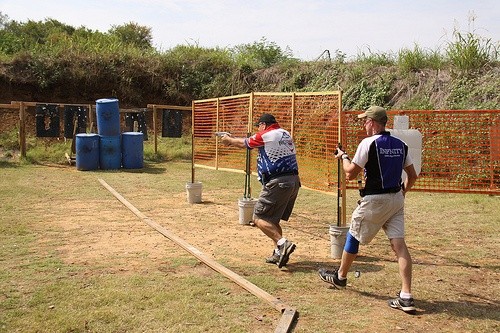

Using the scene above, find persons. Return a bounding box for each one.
[318,105,415,314]
[221,114,301,267]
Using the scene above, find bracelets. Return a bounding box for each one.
[340,152,350,160]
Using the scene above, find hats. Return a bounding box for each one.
[357,106,388,125]
[253,114,276,126]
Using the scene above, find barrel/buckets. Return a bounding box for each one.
[75,131,144,171]
[238,199,258,225]
[329,223,352,259]
[96,98,120,137]
[186,182,202,203]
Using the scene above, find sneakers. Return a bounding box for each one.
[318,268,347,289]
[388,290,416,313]
[266,248,281,263]
[277,240,296,269]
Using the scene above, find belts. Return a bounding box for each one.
[271,170,298,178]
[364,186,401,195]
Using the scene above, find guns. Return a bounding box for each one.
[216,132,231,144]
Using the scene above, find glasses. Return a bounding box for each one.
[361,118,370,123]
[256,124,262,128]
